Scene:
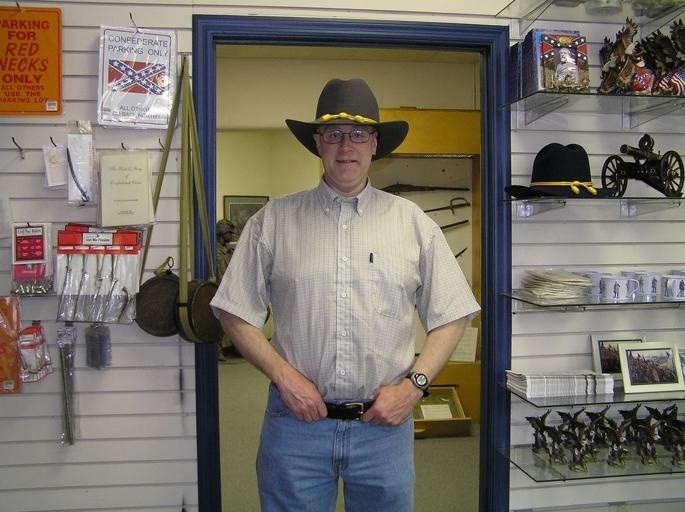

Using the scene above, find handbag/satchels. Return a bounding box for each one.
[134,270,219,343]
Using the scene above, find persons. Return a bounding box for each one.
[210,79,481,511]
[555,47,578,83]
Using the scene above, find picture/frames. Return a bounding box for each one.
[223,194,270,252]
[590,334,646,381]
[617,343,685,394]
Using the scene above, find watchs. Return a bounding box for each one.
[405,372,430,397]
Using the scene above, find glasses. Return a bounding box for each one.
[313,128,379,144]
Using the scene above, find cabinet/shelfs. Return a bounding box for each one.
[494,0,685,483]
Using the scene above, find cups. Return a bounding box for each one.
[586,269,684,302]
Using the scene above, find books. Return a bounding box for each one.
[519,267,594,303]
[505,369,614,399]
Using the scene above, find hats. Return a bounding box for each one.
[504,143,619,199]
[285,79,409,160]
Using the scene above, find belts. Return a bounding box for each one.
[323,400,375,421]
